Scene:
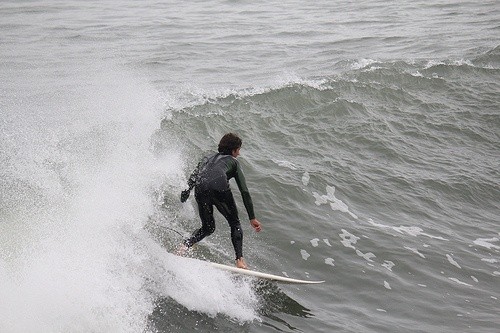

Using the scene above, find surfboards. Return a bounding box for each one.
[170,252,326,284]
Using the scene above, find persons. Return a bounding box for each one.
[176,132,263,270]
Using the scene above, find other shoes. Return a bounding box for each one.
[236,257,249,271]
